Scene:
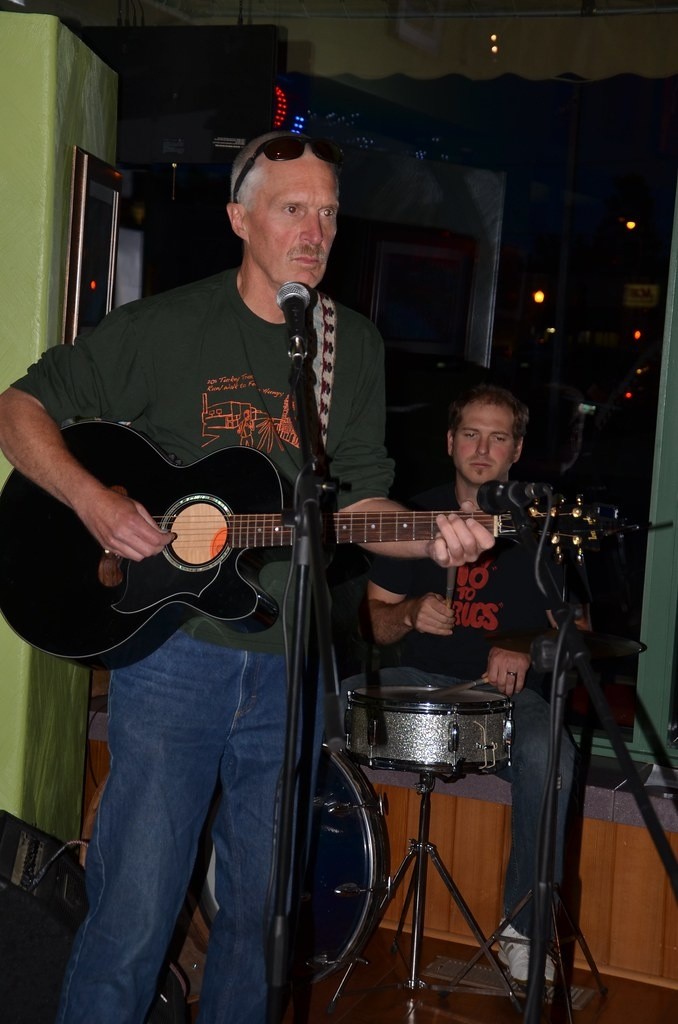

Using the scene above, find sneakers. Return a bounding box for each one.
[496,918,556,982]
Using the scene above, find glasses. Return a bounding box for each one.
[232,135,342,202]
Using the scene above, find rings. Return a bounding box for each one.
[507,672,516,676]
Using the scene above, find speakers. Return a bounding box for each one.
[0,811,189,1024]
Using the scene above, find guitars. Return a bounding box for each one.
[0,418,625,671]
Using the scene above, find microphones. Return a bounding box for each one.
[276,281,311,366]
[476,480,549,514]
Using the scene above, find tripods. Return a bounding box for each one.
[326,664,608,1024]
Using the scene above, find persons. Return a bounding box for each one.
[340,384,590,982]
[0,132,494,1024]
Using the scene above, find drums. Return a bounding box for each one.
[77,743,389,1001]
[344,684,512,774]
